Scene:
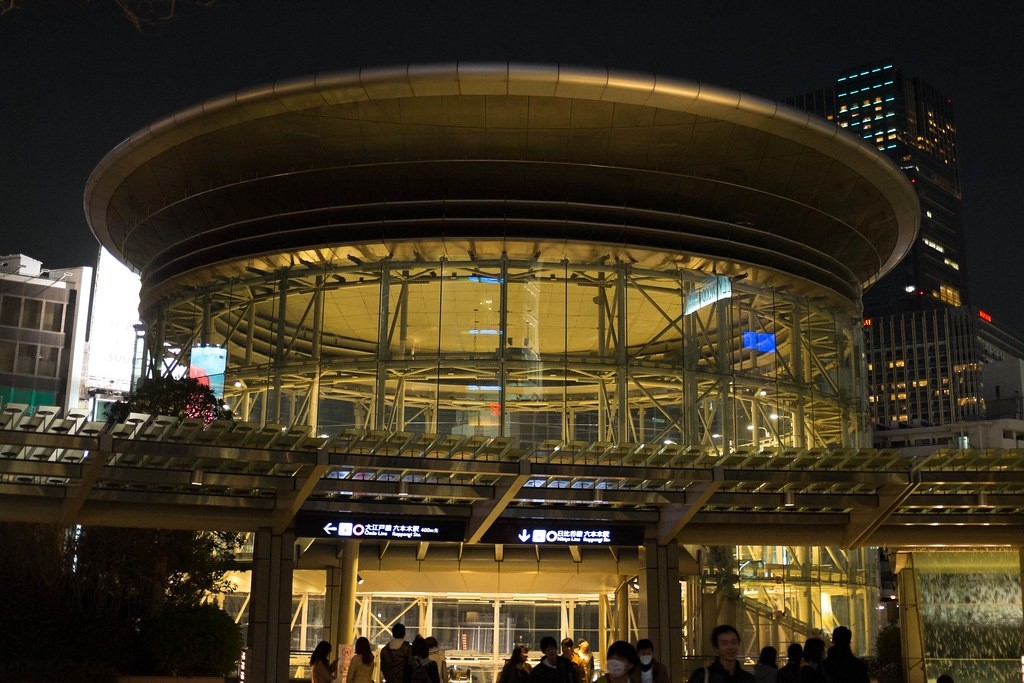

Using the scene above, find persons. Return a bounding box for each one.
[688,625,754,683]
[345,637,374,683]
[309,641,339,683]
[751,625,907,683]
[496,636,669,683]
[380,622,448,683]
